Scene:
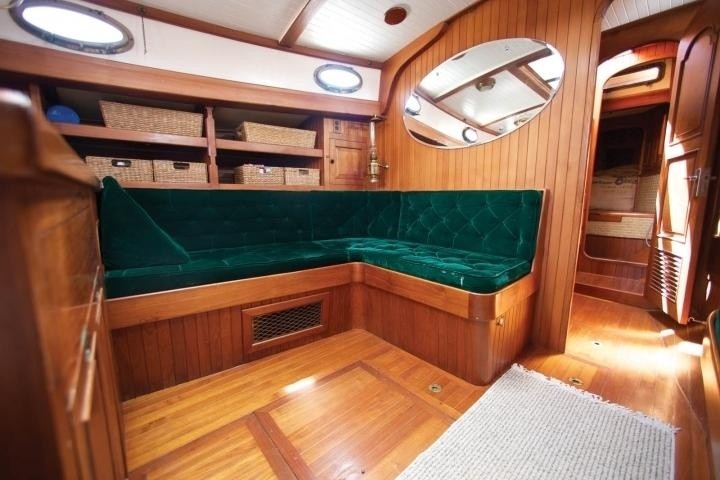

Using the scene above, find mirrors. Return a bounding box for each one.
[401,35,567,150]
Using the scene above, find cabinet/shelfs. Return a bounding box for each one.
[31,78,327,188]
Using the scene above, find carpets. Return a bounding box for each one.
[389,362,678,480]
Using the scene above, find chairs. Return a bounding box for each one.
[95,185,550,401]
